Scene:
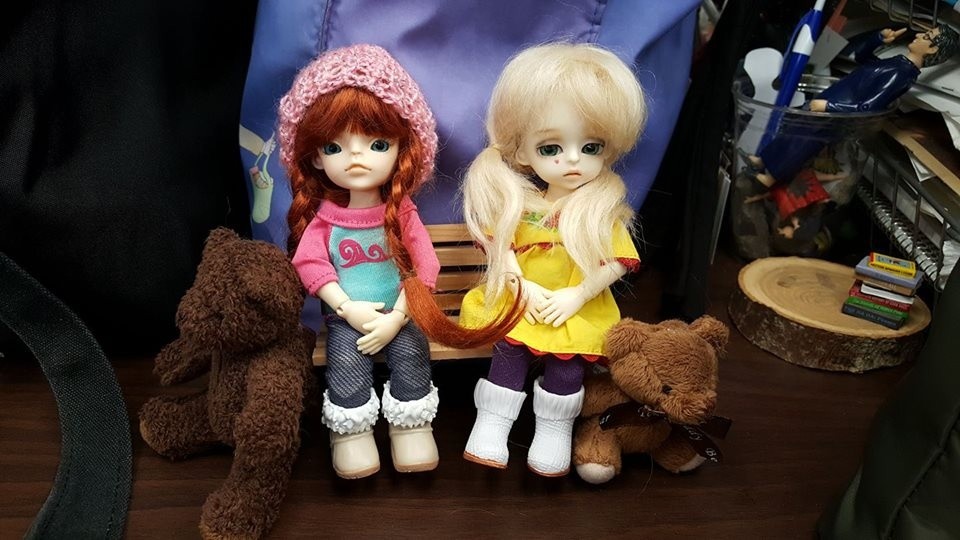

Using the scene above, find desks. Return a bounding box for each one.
[0,249,917,540]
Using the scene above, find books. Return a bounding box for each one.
[841,251,925,331]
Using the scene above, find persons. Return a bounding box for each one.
[739,25,955,194]
[278,44,528,478]
[459,40,649,476]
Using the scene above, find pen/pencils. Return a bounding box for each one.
[746,0,826,182]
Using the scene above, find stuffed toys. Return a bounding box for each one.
[572,315,734,484]
[142,227,317,540]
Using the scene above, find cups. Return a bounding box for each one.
[731,74,900,264]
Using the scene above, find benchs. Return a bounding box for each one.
[309,222,494,371]
